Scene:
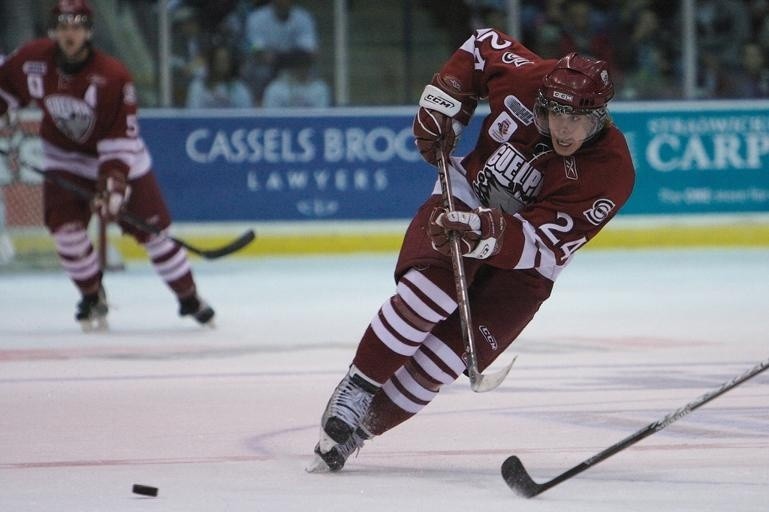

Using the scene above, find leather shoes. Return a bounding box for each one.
[413,72,479,168]
[427,205,505,260]
[90,160,132,225]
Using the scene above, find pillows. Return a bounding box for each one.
[49,1,93,28]
[533,52,615,143]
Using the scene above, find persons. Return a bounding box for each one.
[443,3,766,100]
[147,3,327,108]
[0,2,214,325]
[314,27,637,472]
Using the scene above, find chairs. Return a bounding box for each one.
[76,286,108,320]
[314,423,376,470]
[321,375,375,445]
[180,293,214,323]
